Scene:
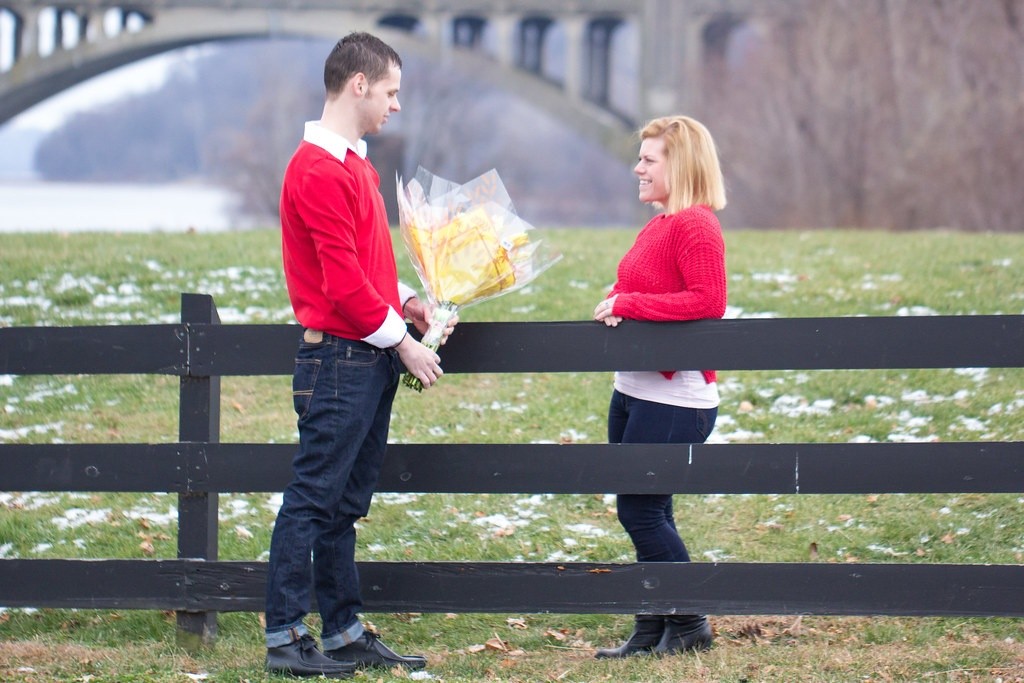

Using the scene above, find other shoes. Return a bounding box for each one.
[634,615,712,659]
[595,615,665,660]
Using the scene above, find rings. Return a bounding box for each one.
[605,302,609,308]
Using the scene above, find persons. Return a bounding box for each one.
[593,115,726,660]
[264,32,460,680]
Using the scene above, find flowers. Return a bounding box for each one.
[397,172,557,391]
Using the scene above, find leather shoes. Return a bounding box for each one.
[264,634,357,677]
[324,629,428,671]
